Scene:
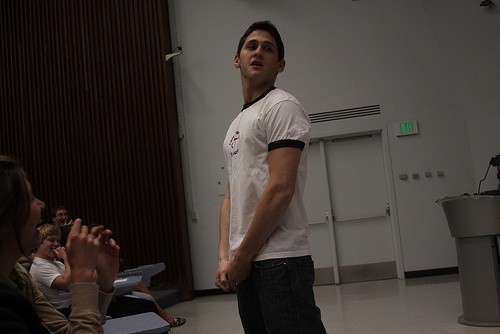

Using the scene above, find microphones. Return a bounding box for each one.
[478,156,495,194]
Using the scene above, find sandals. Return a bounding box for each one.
[168,316,186,327]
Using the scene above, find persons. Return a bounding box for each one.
[215,21,328,333]
[0,153,121,334]
[28,222,160,320]
[47,207,186,329]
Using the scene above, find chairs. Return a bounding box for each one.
[17,249,172,334]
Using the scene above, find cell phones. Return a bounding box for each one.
[60,224,73,248]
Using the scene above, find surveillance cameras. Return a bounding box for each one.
[177,40,183,50]
